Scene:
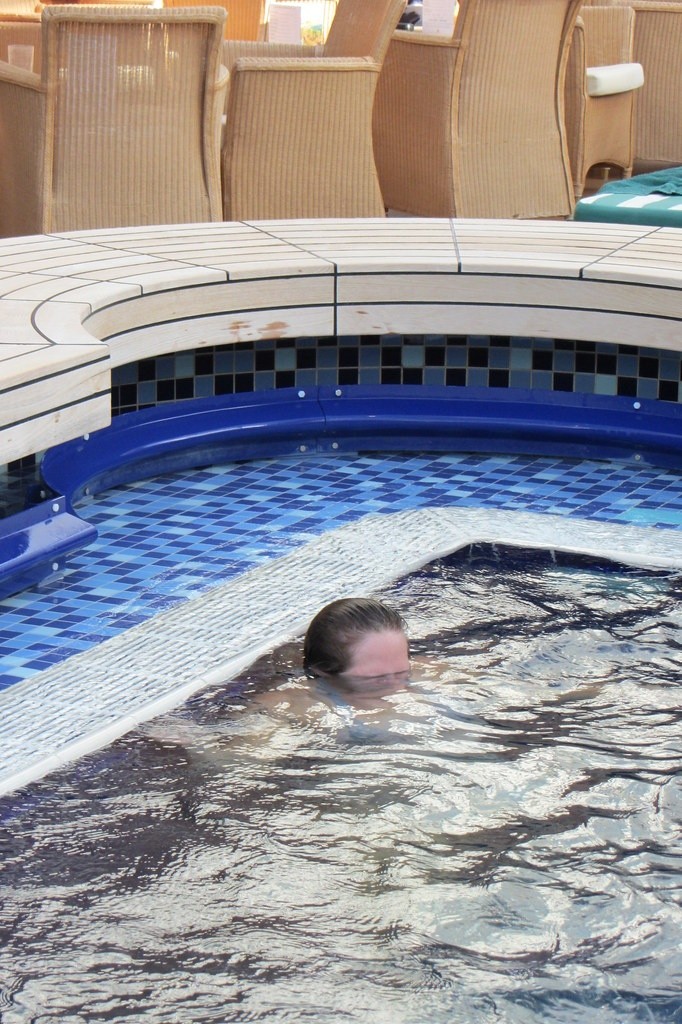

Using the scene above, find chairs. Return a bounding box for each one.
[0,0,682,239]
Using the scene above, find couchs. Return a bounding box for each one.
[573,165,682,231]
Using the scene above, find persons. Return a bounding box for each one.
[303,598,410,676]
